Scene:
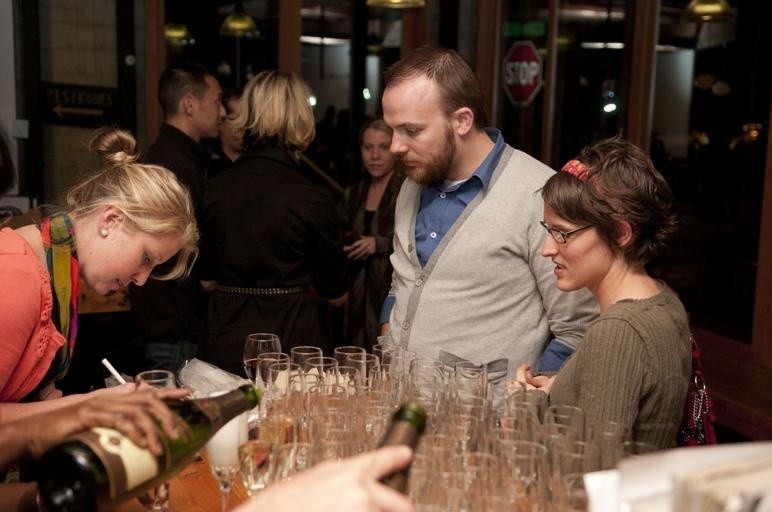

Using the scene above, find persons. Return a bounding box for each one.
[130,43,404,382]
[226,444,419,512]
[375,39,597,401]
[505,136,697,476]
[1,386,194,511]
[2,126,201,431]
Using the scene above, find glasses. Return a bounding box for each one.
[540,218,595,246]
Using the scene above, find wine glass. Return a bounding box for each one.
[204,331,661,512]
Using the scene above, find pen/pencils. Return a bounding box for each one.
[100,358,126,384]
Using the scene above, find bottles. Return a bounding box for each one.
[36,384,266,512]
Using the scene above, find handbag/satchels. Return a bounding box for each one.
[679,384,717,448]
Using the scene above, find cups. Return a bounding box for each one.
[137,370,179,393]
